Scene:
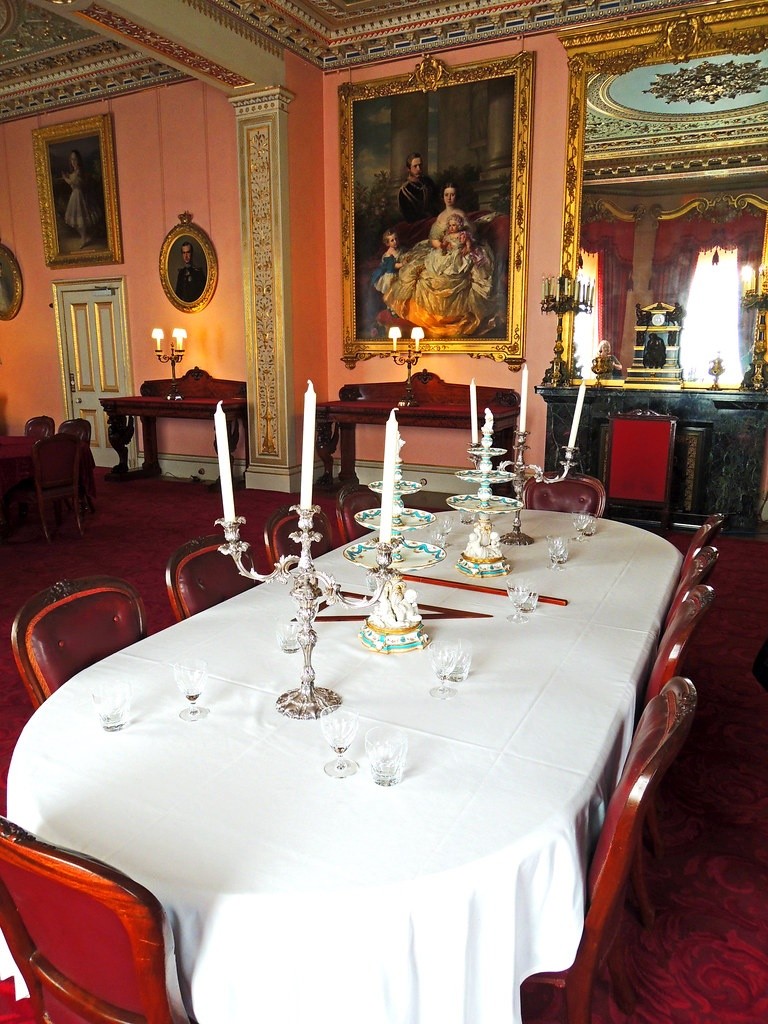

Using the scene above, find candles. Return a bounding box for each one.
[213,400,236,523]
[740,263,768,291]
[539,273,597,303]
[379,406,400,542]
[300,379,317,506]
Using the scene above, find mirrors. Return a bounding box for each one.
[560,142,768,389]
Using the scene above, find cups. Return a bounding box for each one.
[276,614,303,654]
[519,584,538,614]
[91,678,132,733]
[363,724,408,786]
[459,511,476,526]
[582,513,598,536]
[550,539,568,564]
[431,529,445,549]
[447,637,472,682]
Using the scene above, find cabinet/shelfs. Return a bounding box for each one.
[530,385,768,539]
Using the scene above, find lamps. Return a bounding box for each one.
[151,328,187,400]
[389,327,424,406]
[469,364,587,448]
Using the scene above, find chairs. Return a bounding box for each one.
[0,416,384,1024]
[518,471,724,1024]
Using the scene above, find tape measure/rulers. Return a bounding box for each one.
[291,590,494,623]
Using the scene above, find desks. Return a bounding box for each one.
[98,367,248,493]
[0,435,45,542]
[314,370,523,499]
[0,500,686,1024]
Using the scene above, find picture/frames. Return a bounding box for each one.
[0,244,24,321]
[31,113,125,270]
[338,51,538,369]
[158,212,220,314]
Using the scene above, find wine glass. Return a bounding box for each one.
[437,516,453,547]
[546,534,566,572]
[571,510,590,544]
[174,657,210,722]
[319,705,359,778]
[428,640,459,701]
[507,575,531,625]
